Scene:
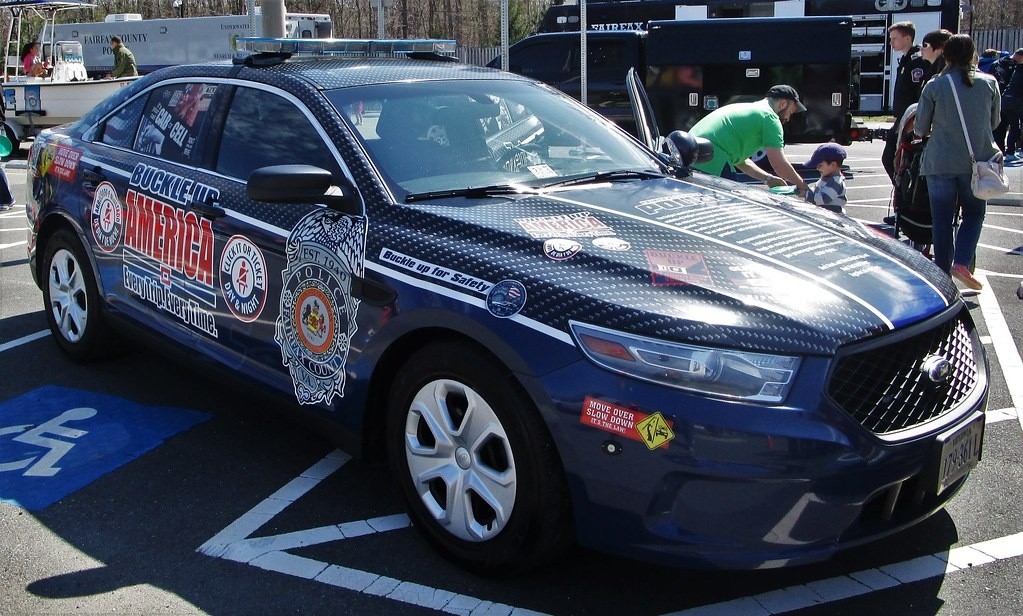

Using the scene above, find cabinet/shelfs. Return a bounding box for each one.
[850,14,888,107]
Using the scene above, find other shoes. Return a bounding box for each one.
[883,215,896,225]
[949,264,983,290]
[1019,151,1023,157]
[1011,244,1023,255]
[1006,154,1020,161]
[0,201,16,211]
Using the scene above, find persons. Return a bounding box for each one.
[975,47,1023,162]
[913,33,1003,290]
[0,76,16,213]
[344,100,364,126]
[917,28,954,109]
[881,21,930,226]
[797,142,847,216]
[688,85,810,202]
[20,42,50,77]
[103,36,138,80]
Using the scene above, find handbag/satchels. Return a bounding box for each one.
[971,152,1010,200]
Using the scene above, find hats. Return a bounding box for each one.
[766,85,807,113]
[803,143,847,168]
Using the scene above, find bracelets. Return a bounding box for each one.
[764,173,773,184]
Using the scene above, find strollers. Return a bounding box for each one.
[893,102,976,275]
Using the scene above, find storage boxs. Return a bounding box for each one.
[867,27,884,36]
[852,27,866,35]
[859,94,882,111]
[851,44,884,56]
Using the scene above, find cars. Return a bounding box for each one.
[25,36,994,583]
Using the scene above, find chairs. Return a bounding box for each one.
[367,99,439,183]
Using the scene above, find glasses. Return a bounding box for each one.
[923,42,930,49]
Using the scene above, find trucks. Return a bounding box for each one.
[34,4,335,80]
[486,0,861,146]
